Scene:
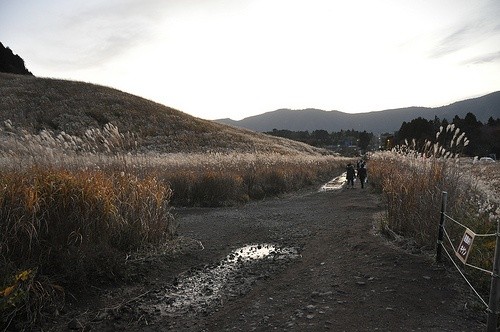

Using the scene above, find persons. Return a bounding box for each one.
[356,163,366,189]
[355,159,369,183]
[346,163,356,189]
